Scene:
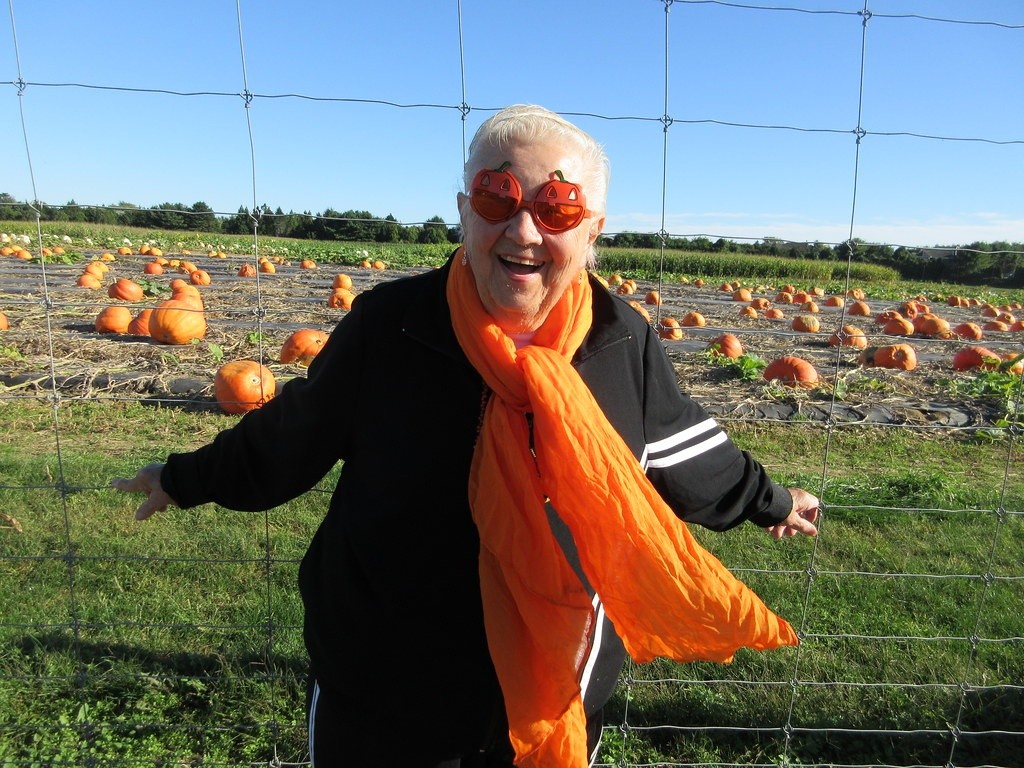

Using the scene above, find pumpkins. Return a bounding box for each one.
[1,232,1024,413]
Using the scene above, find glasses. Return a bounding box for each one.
[467,161,596,234]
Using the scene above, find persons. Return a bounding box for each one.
[112,105,825,768]
